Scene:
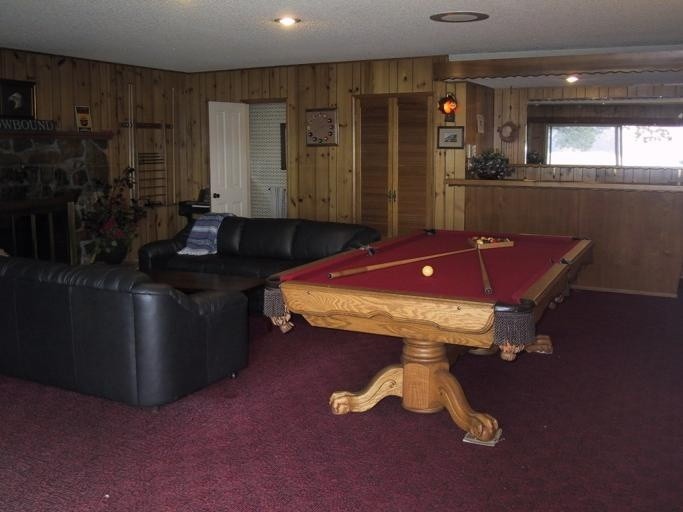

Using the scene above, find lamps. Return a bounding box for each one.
[438,91,458,122]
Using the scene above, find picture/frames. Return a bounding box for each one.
[0,77,38,120]
[436,125,465,150]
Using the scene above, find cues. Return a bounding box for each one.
[474,243,493,296]
[328,248,477,278]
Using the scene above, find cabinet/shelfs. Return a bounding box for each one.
[351,91,435,242]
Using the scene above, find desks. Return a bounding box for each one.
[258,228,596,446]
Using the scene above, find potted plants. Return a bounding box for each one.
[467,148,509,180]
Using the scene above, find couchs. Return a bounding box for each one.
[1,255,250,409]
[139,216,378,276]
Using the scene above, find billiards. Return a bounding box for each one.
[422,266,433,277]
[472,236,511,244]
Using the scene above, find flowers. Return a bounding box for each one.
[77,164,163,250]
